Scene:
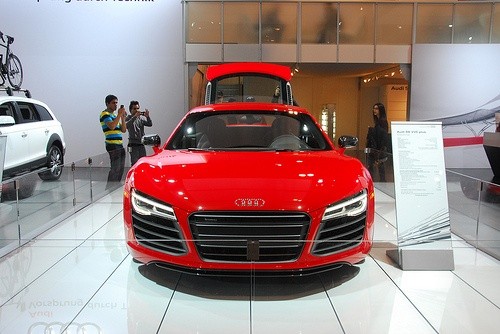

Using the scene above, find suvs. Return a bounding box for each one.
[0,87,66,186]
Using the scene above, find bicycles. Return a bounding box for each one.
[0,31,24,89]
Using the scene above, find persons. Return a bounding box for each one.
[239,95,267,124]
[212,91,237,125]
[125,100,152,167]
[367,103,388,182]
[99,94,126,189]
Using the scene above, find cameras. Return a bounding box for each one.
[140,112,145,115]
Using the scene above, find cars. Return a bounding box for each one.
[122,64,375,280]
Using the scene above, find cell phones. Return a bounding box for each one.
[121,105,124,108]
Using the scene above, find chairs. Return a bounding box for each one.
[196,116,226,148]
[271,116,301,146]
